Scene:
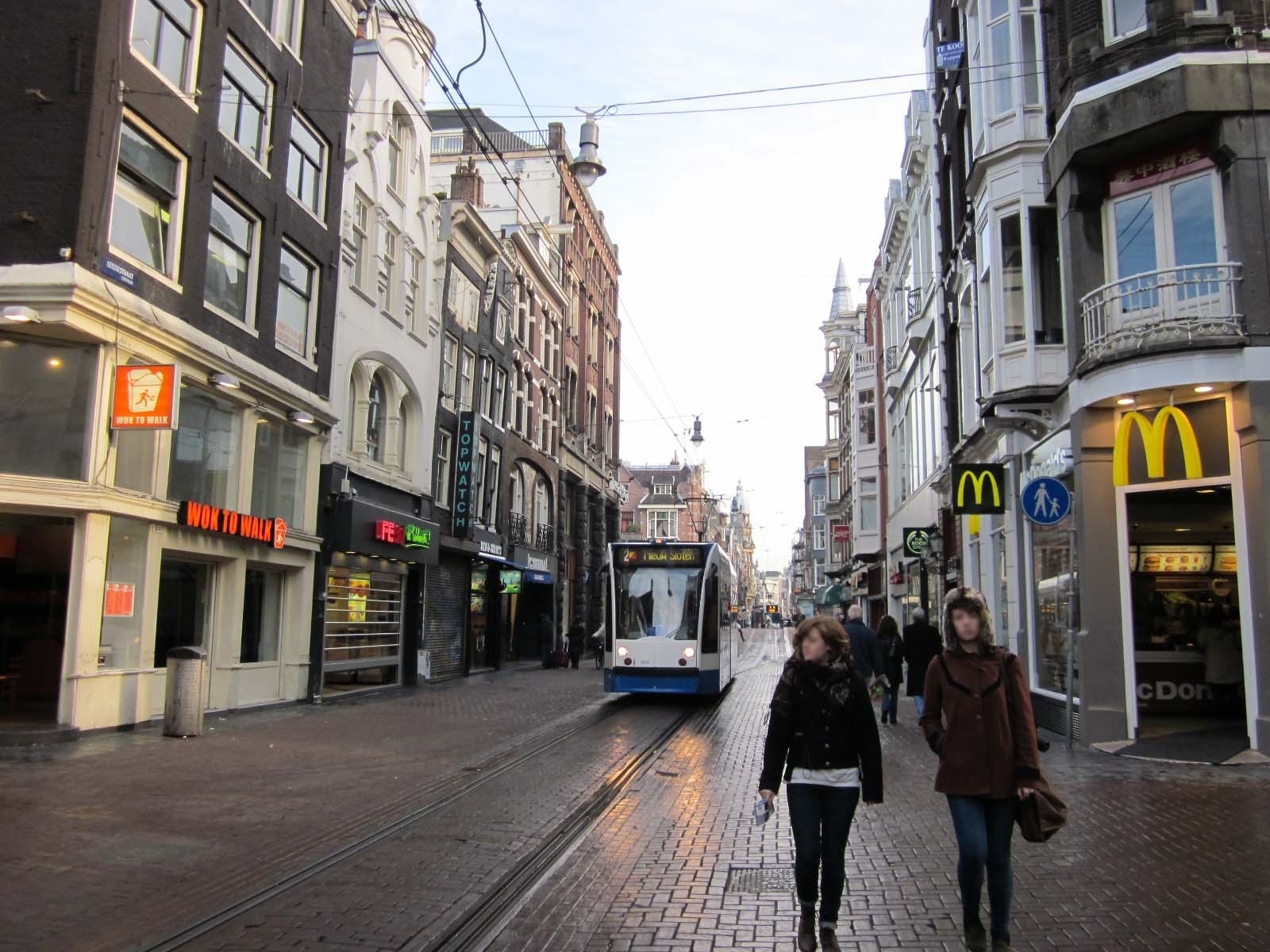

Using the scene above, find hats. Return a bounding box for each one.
[943,585,993,651]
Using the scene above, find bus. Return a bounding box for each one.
[599,494,738,699]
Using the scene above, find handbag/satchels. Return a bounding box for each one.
[1015,776,1069,843]
[870,679,884,701]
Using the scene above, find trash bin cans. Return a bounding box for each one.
[163,646,208,738]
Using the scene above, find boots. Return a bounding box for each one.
[797,903,818,952]
[819,927,841,952]
[890,707,898,724]
[881,710,888,723]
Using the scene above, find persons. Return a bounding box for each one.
[568,616,585,669]
[903,607,943,727]
[876,616,904,725]
[592,623,606,646]
[737,609,845,641]
[759,616,883,952]
[842,604,887,689]
[924,588,1051,952]
[1197,606,1244,728]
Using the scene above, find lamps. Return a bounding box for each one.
[208,375,241,389]
[571,109,606,186]
[1,305,45,327]
[920,523,957,572]
[288,412,315,425]
[691,415,704,447]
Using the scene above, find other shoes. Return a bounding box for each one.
[964,926,987,952]
[992,939,1017,952]
